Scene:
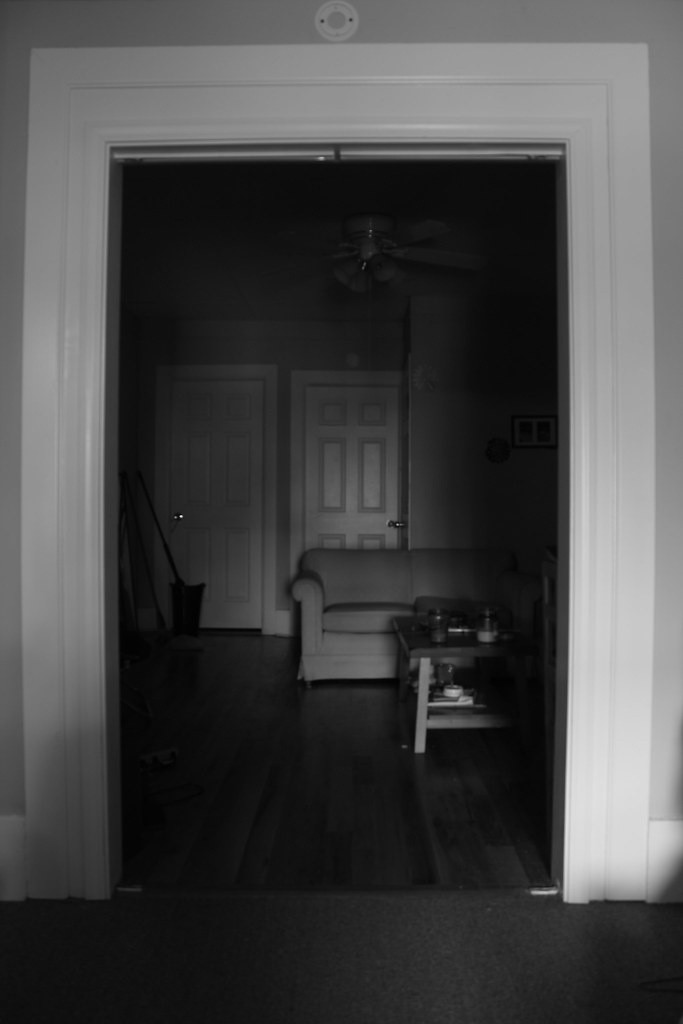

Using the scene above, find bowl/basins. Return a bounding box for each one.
[443,685,464,698]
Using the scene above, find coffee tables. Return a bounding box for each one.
[389,616,537,753]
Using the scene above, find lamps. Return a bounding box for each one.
[334,249,406,291]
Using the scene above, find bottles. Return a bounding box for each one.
[427,608,451,642]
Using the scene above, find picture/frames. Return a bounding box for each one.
[510,414,558,449]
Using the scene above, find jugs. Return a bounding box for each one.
[474,605,503,644]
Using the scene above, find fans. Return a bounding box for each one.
[259,214,488,286]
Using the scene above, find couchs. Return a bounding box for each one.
[291,545,543,679]
